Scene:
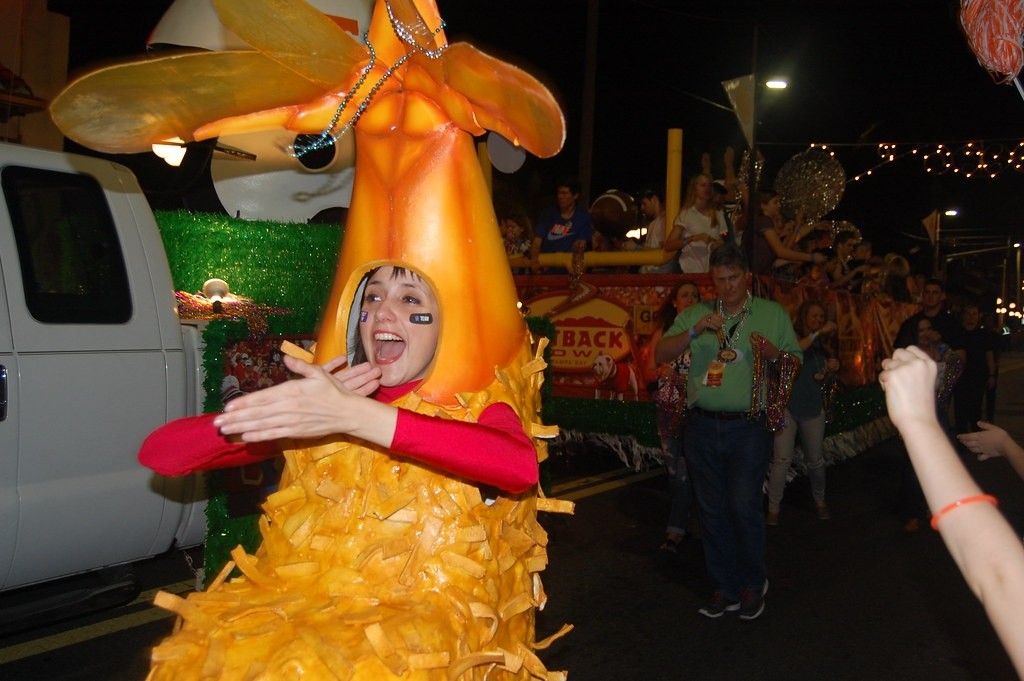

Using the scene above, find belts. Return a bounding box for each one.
[694,406,752,420]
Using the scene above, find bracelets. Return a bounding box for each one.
[809,331,820,340]
[687,326,697,341]
[931,495,999,531]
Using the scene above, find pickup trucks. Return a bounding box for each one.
[2,143,209,610]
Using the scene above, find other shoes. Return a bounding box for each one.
[767,511,780,525]
[658,539,681,556]
[905,518,919,534]
[818,507,832,521]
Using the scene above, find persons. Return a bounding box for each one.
[496,173,997,548]
[957,421,1023,480]
[878,344,1023,678]
[651,243,805,623]
[138,264,539,497]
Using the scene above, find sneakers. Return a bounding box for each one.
[697,592,741,618]
[739,576,768,620]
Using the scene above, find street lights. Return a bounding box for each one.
[747,68,790,269]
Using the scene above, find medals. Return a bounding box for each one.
[718,347,738,364]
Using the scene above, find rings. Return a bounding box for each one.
[708,317,711,322]
